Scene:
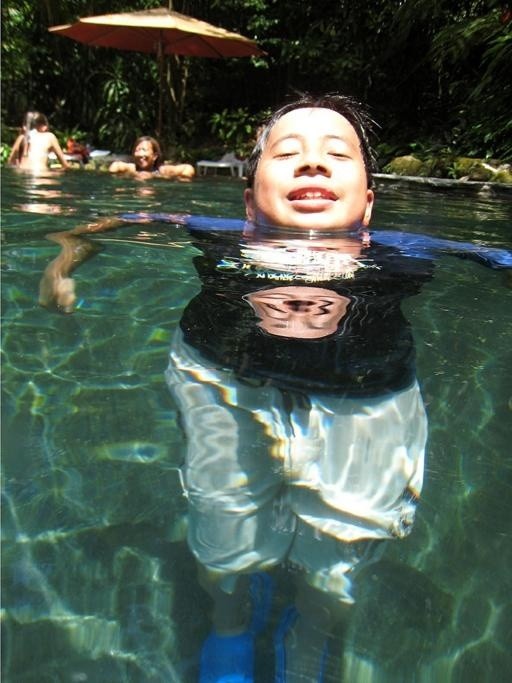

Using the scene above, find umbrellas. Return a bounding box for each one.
[48,6,270,142]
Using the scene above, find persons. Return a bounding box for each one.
[6,108,195,183]
[36,94,512,683]
